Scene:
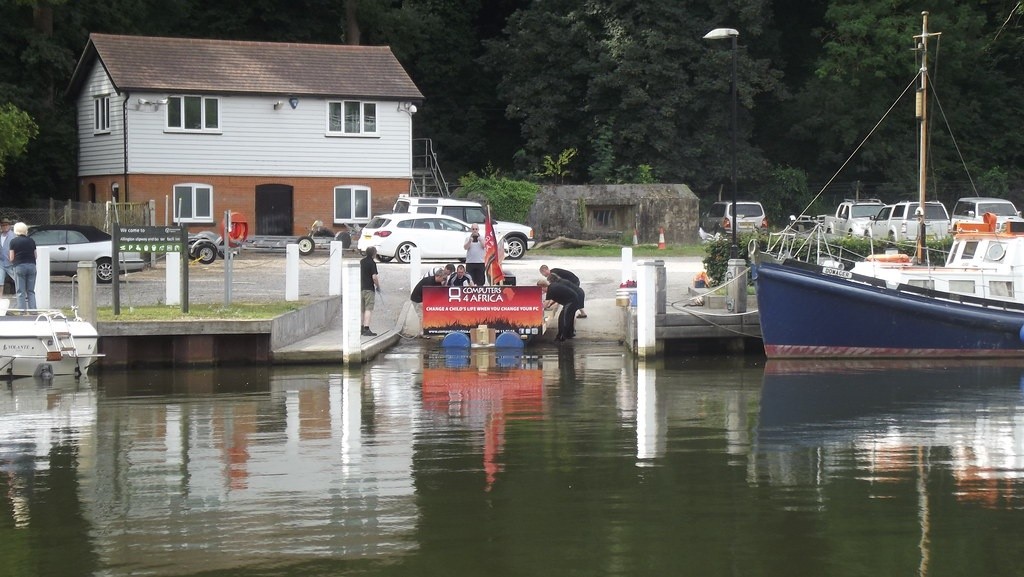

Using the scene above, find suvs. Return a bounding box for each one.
[392,193,535,260]
[865,200,951,245]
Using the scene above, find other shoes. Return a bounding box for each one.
[577,314,587,318]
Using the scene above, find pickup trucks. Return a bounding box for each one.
[823,198,887,238]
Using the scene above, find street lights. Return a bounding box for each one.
[703,27,739,255]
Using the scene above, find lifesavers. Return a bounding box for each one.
[867,253,909,263]
[229,221,246,244]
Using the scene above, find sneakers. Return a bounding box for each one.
[363,328,377,336]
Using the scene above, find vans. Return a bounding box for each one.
[701,201,768,239]
[949,196,1024,242]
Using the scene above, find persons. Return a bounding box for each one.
[361,246,380,335]
[9,222,37,314]
[464,223,485,286]
[411,263,473,339]
[537,265,587,342]
[0,219,28,299]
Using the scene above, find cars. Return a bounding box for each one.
[26,224,144,284]
[357,212,510,264]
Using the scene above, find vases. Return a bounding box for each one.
[624,288,637,309]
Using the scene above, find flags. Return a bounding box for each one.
[485,217,505,284]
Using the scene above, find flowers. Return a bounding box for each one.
[620,279,637,288]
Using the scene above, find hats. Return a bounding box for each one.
[0,218,12,224]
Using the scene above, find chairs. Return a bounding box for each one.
[424,223,430,229]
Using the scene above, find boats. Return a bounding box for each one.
[0,299,106,379]
[753,10,1024,361]
[751,360,1024,577]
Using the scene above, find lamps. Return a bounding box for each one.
[274,101,284,111]
[398,101,418,116]
[137,97,169,111]
